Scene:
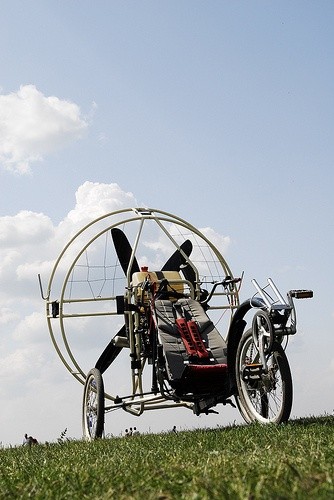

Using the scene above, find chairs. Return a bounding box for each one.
[150,298,227,389]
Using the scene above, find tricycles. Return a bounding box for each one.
[37,208,316,435]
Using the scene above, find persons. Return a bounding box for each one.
[132,427,139,437]
[172,425,177,433]
[125,429,129,436]
[22,434,37,446]
[129,427,133,436]
